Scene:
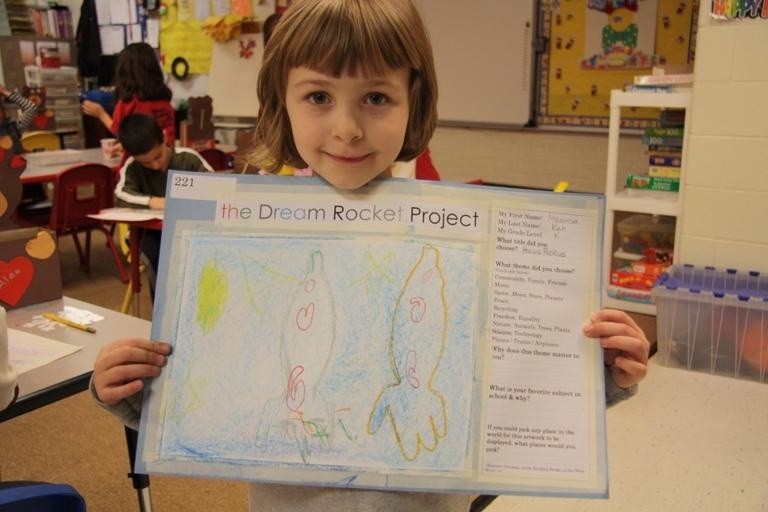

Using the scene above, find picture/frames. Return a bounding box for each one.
[524,0,694,127]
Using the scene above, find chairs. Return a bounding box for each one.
[21,95,258,314]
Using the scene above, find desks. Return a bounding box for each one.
[475,351,768,512]
[0,296,154,512]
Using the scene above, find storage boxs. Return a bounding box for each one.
[655,263,768,384]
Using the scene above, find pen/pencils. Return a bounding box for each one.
[39,311,97,334]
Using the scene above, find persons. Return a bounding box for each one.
[86,1,653,511]
[0,85,37,146]
[81,39,175,180]
[110,113,215,305]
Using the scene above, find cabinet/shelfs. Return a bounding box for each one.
[601,89,691,317]
[38,67,85,150]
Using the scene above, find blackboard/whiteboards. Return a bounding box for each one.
[123,0,539,129]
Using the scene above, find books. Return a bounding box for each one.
[3,326,86,399]
[28,4,74,38]
[624,109,685,193]
